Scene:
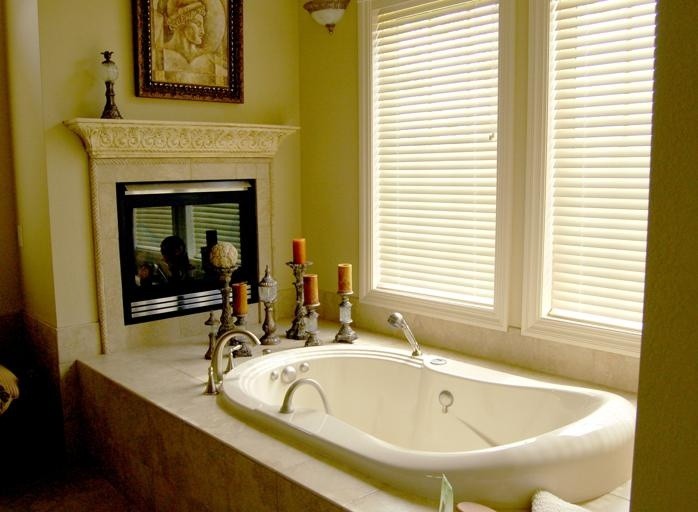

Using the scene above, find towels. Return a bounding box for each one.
[531,490,590,512]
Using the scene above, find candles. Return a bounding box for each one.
[199,229,218,267]
[232,281,248,315]
[293,238,306,265]
[338,264,352,293]
[303,274,318,304]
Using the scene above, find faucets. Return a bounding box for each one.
[209,329,261,383]
[387,312,424,357]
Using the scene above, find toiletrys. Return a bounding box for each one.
[438,471,454,512]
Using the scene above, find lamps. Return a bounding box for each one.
[303,0,351,36]
[258,266,281,344]
[98,50,123,119]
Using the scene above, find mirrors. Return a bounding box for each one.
[115,178,261,327]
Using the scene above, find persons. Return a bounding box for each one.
[156,0,208,60]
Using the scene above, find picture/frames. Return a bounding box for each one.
[132,0,244,103]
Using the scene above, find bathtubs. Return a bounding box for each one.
[219,343,638,512]
